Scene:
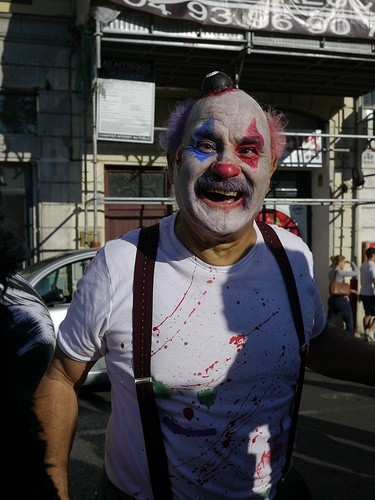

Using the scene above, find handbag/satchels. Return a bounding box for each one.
[329,270,350,295]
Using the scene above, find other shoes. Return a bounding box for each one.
[363,329,375,343]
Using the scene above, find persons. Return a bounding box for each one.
[326,255,354,334]
[0,206,56,500]
[27,70,327,500]
[359,246,375,342]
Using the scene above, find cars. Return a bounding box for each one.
[18,249,110,393]
